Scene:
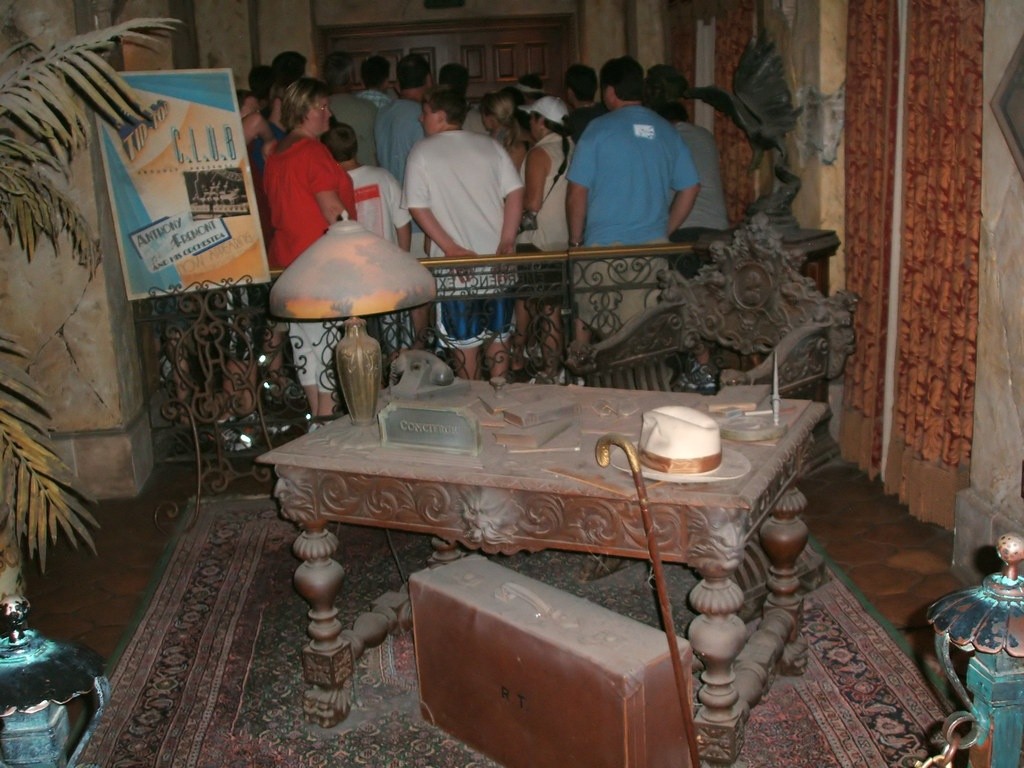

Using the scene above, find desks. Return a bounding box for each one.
[255,379,831,768]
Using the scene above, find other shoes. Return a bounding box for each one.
[206,418,252,452]
[529,370,587,388]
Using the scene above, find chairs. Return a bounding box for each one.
[568,211,858,624]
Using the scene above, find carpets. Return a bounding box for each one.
[66,494,968,768]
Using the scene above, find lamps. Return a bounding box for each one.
[269,210,438,425]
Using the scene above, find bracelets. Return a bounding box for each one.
[568,240,584,246]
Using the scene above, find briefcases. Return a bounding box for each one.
[407,552,695,768]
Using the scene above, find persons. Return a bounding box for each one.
[263,75,357,427]
[140,50,732,451]
[512,98,574,376]
[565,56,700,341]
[399,83,527,380]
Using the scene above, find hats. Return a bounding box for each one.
[518,96,570,126]
[609,405,751,483]
